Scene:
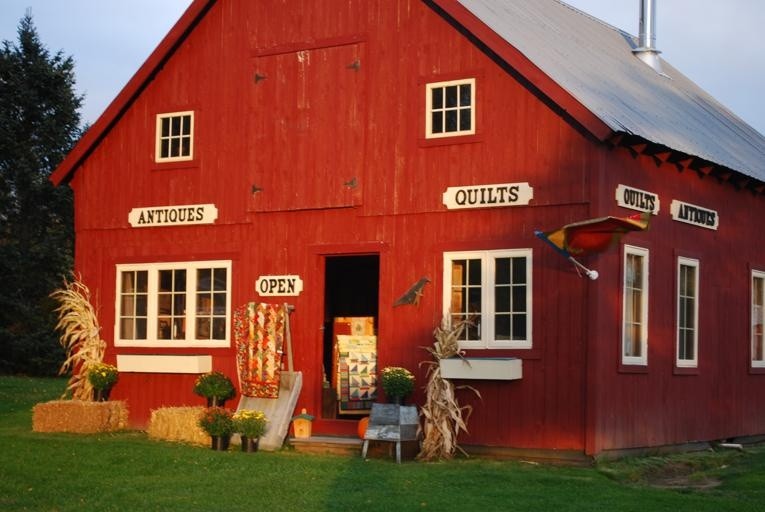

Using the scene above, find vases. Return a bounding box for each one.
[87,359,119,391]
[192,369,236,402]
[231,408,270,438]
[380,366,416,397]
[194,406,235,437]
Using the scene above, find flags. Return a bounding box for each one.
[389,394,404,406]
[212,435,229,451]
[93,386,109,402]
[207,395,219,408]
[241,435,258,453]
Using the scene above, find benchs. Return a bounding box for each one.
[537,209,655,260]
